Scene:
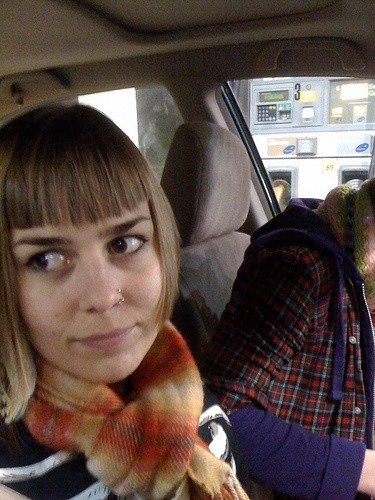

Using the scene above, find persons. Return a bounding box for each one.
[201,177,375,500]
[1,103,251,500]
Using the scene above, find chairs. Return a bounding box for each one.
[160,117,254,367]
